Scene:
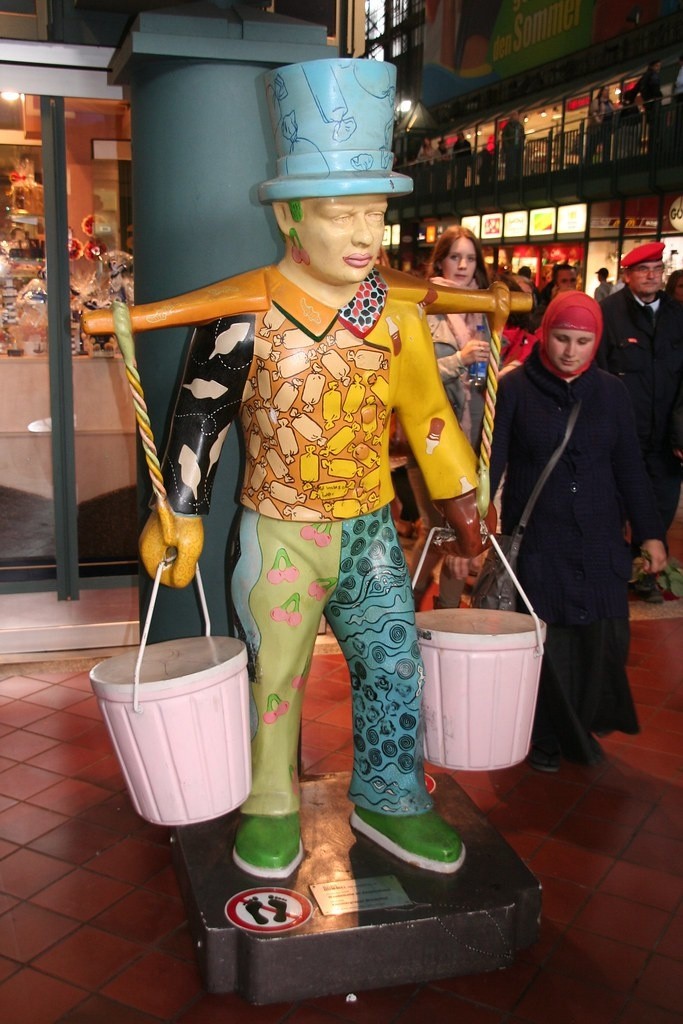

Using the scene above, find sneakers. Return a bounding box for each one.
[634,582,664,603]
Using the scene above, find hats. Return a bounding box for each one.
[596,268,608,276]
[541,290,603,335]
[620,242,665,269]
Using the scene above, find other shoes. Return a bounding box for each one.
[527,749,560,772]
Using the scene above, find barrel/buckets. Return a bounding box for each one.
[411,527,546,771]
[89,555,252,825]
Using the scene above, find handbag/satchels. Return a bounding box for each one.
[471,534,517,612]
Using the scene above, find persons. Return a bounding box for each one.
[477,290,669,770]
[417,51,683,192]
[596,244,683,603]
[143,58,498,878]
[374,227,577,611]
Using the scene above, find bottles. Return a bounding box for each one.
[4,302,25,357]
[468,325,489,385]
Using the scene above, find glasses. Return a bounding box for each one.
[625,267,665,274]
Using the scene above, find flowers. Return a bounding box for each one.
[628,546,683,605]
